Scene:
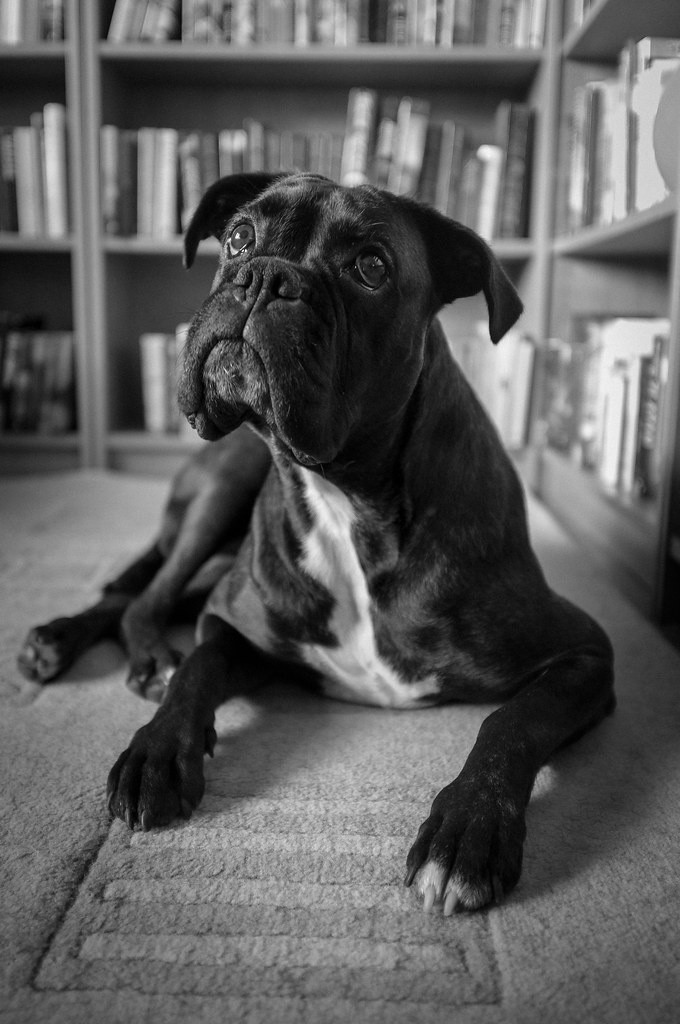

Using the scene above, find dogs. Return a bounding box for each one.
[15,170,617,915]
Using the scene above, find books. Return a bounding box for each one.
[1,317,671,517]
[0,0,680,239]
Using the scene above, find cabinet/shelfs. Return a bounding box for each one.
[79,0,565,481]
[0,0,91,474]
[525,0,680,622]
